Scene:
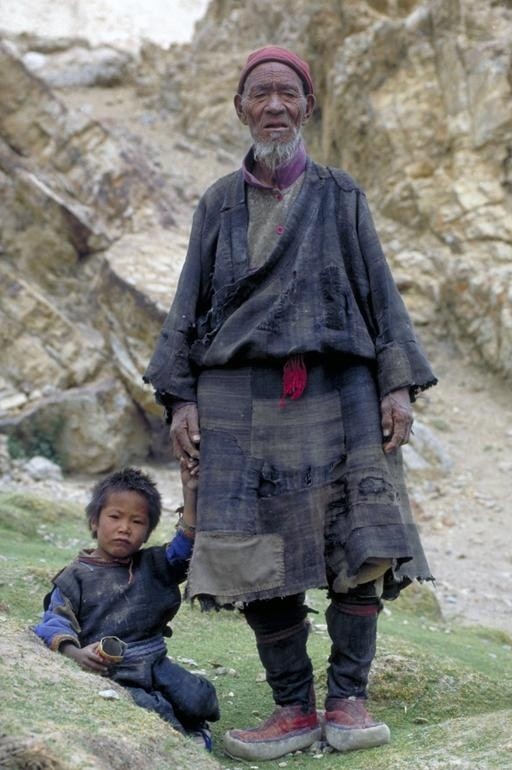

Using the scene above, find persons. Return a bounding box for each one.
[30,448,224,755]
[144,42,442,761]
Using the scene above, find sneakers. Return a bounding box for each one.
[191,721,211,756]
[320,697,394,749]
[223,698,323,763]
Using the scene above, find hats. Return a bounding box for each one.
[235,44,314,96]
